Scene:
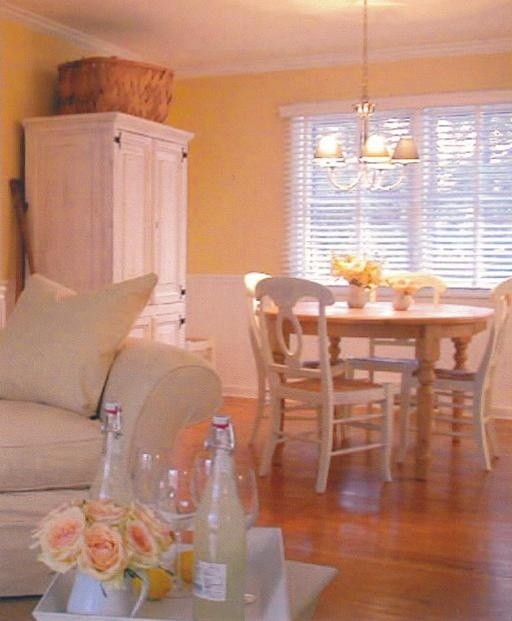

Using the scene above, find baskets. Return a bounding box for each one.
[54,52,175,123]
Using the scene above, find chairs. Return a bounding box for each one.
[341,272,451,466]
[242,270,369,457]
[398,278,512,477]
[253,275,394,497]
[1,275,224,605]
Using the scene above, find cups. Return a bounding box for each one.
[66,559,150,619]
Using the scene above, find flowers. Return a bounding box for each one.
[326,252,388,287]
[27,495,173,587]
[391,273,448,304]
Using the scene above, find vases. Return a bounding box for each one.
[66,570,130,619]
[390,295,413,311]
[346,282,367,310]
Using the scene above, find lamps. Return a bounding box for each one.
[311,1,422,196]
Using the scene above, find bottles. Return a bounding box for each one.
[85,400,261,621]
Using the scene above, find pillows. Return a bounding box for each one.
[2,269,161,419]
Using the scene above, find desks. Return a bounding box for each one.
[254,301,496,477]
[185,334,215,371]
[31,548,337,620]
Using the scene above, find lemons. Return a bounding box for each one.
[177,549,196,584]
[132,567,172,601]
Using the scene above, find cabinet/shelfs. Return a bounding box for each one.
[125,308,190,352]
[18,112,197,308]
